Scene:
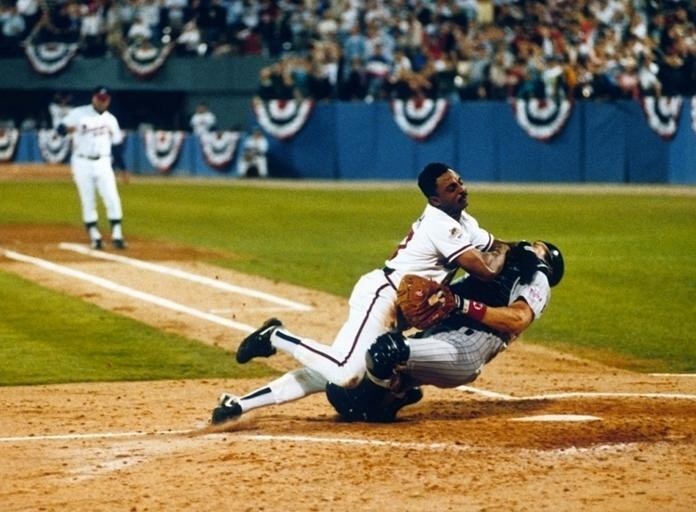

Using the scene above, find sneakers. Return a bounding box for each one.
[88,223,125,240]
[236,317,282,364]
[212,392,242,422]
[325,381,423,421]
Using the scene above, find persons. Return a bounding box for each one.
[54,84,128,250]
[0,0,695,103]
[207,161,515,427]
[190,104,217,133]
[324,239,566,426]
[236,126,269,178]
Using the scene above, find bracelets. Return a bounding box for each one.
[466,299,487,323]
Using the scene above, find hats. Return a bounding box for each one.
[92,86,111,100]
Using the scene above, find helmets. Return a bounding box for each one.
[535,240,563,287]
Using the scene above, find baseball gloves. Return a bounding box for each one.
[397,276,453,330]
